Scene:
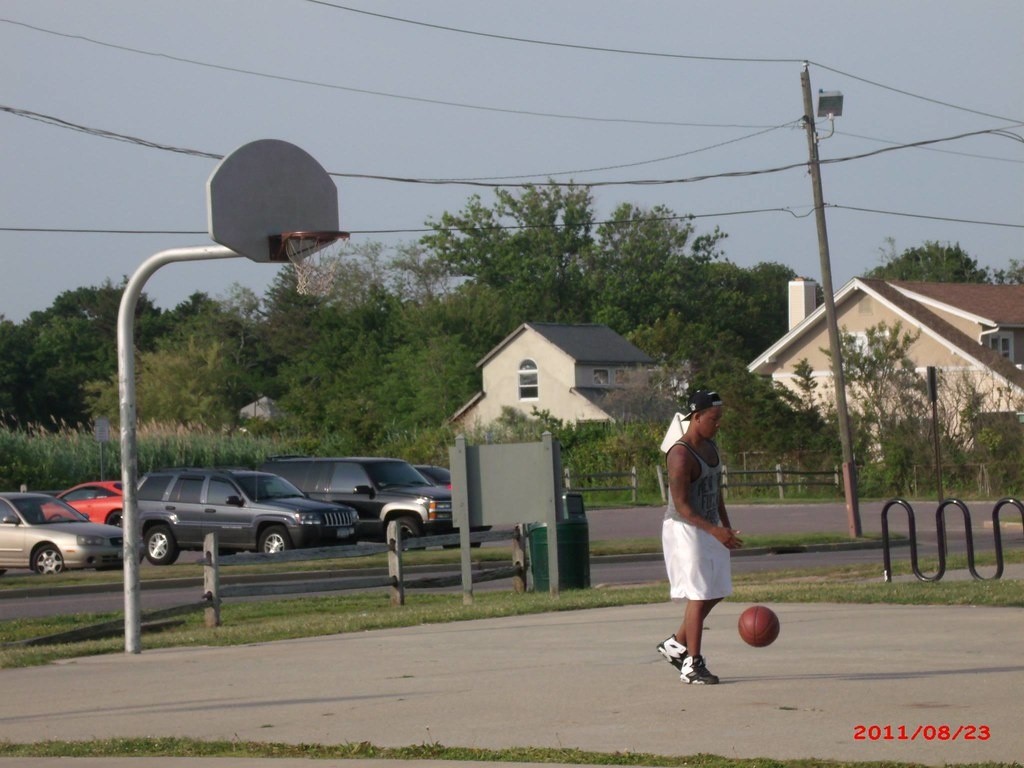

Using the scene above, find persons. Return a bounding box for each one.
[658,388,747,686]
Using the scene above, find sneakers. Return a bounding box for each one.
[657,634,688,672]
[679,655,719,685]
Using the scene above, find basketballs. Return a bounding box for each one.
[737,605,780,647]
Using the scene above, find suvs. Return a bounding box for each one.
[136,467,361,565]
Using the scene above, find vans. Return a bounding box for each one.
[256,456,481,551]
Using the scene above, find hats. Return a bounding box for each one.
[680,389,723,421]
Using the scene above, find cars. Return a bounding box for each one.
[0,493,146,576]
[412,465,493,531]
[41,480,123,528]
